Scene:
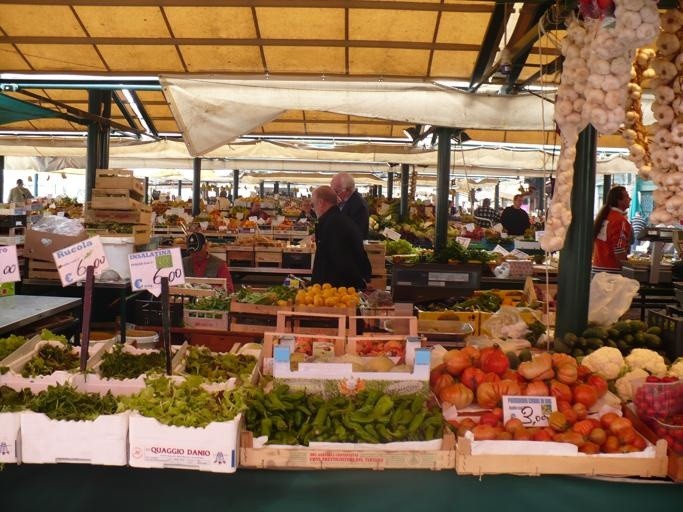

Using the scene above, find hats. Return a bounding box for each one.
[186,232,207,254]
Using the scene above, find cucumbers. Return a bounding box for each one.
[553,320,662,363]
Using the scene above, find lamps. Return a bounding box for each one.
[388,162,399,167]
[500,48,513,75]
[403,128,421,144]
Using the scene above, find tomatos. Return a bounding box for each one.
[634,376,683,456]
[430,346,647,454]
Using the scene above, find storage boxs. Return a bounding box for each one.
[22,226,88,262]
[362,242,386,290]
[0,339,262,473]
[391,260,481,301]
[415,289,542,341]
[0,210,29,227]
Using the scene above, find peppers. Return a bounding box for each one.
[245,384,444,446]
[454,293,502,312]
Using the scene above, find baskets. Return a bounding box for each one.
[647,306,682,356]
[132,295,197,330]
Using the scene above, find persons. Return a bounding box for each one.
[447,185,647,278]
[310,185,372,333]
[220,188,228,197]
[181,231,234,297]
[330,172,370,288]
[5,179,34,205]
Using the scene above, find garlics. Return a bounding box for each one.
[539,0,683,252]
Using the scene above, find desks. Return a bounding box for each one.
[21,279,130,343]
[0,295,82,346]
[227,251,282,269]
[198,233,308,242]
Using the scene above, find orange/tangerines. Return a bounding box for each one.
[506,349,533,370]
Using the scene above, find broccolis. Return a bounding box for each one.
[581,347,683,402]
